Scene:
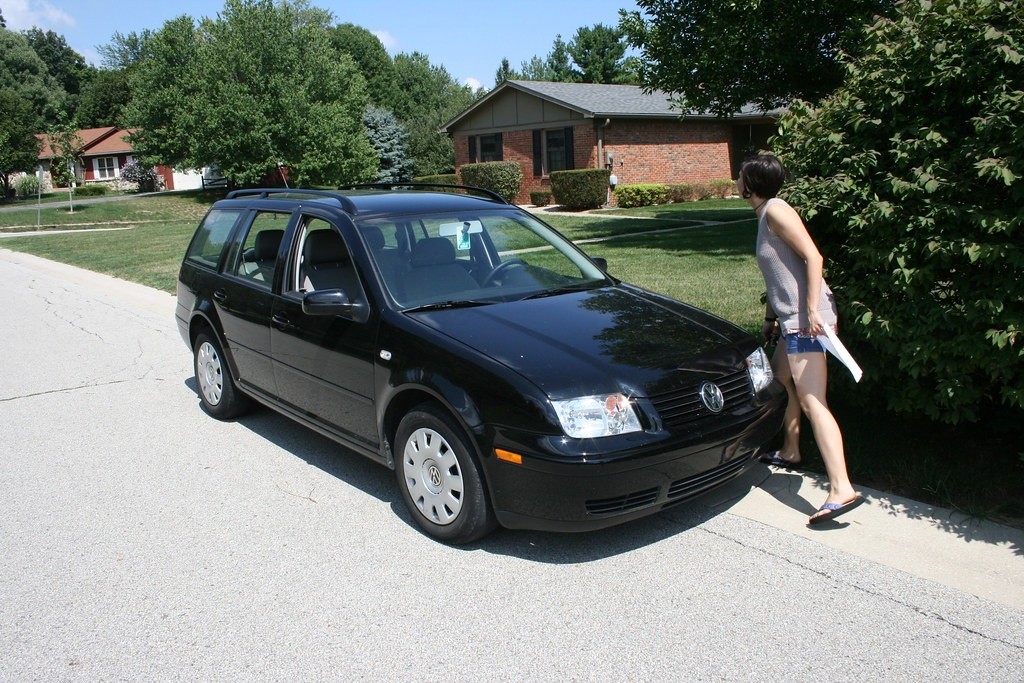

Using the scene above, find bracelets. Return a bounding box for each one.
[765,318,776,322]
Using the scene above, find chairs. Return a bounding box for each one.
[360,227,386,249]
[240,229,284,288]
[405,237,480,301]
[299,229,361,305]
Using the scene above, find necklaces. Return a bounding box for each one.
[754,200,766,212]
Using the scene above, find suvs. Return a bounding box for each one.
[176,182,788,546]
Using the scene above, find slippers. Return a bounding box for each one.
[759,450,803,469]
[809,496,865,525]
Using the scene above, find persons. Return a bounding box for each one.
[609,171,618,192]
[737,155,865,524]
